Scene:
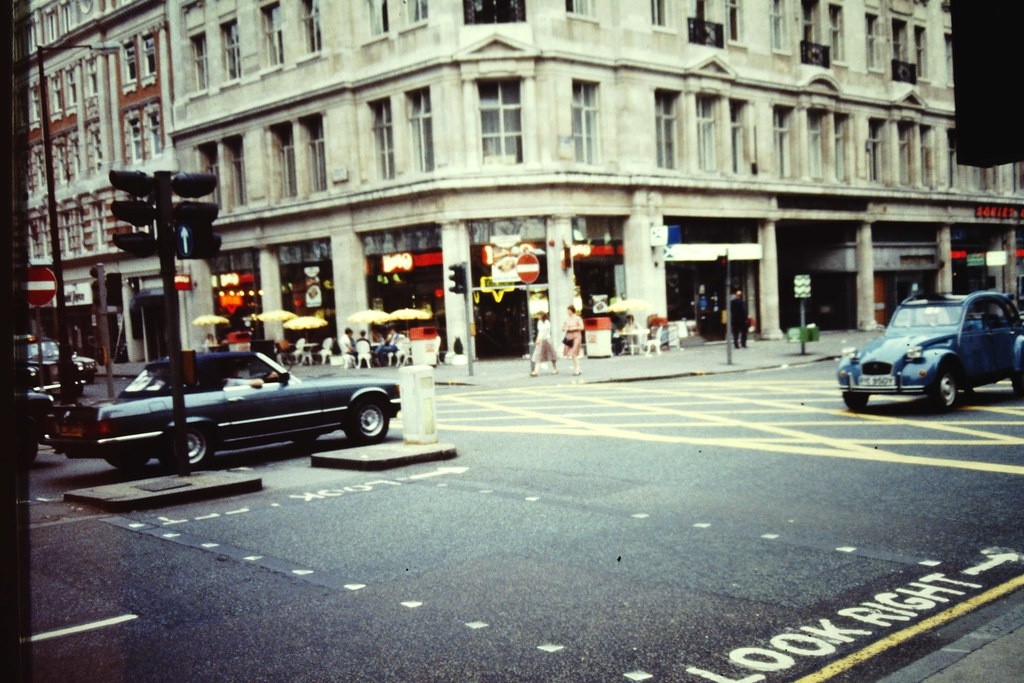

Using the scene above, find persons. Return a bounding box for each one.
[355,327,373,367]
[530,312,560,376]
[609,315,642,355]
[702,290,726,340]
[340,327,356,366]
[370,327,406,366]
[730,291,749,349]
[203,334,216,352]
[223,365,264,387]
[561,304,584,376]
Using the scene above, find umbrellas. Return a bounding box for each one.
[257,311,297,321]
[192,314,229,326]
[284,316,329,331]
[384,306,431,322]
[346,308,387,324]
[605,298,657,312]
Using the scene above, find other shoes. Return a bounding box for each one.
[549,367,561,375]
[573,368,584,376]
[530,368,539,377]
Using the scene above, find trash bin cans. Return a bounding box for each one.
[584,317,613,358]
[227,331,252,352]
[410,326,437,368]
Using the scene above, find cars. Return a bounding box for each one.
[51,348,402,475]
[13,331,99,473]
[835,287,1024,416]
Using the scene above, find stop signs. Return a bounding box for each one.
[515,253,540,283]
[27,264,57,305]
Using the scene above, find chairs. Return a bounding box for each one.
[647,325,663,355]
[293,338,411,366]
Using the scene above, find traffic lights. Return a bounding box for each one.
[172,171,222,260]
[109,169,171,259]
[448,262,469,294]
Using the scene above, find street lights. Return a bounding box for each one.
[34,40,123,350]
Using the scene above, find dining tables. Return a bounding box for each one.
[620,332,642,354]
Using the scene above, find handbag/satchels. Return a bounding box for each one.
[563,336,575,347]
